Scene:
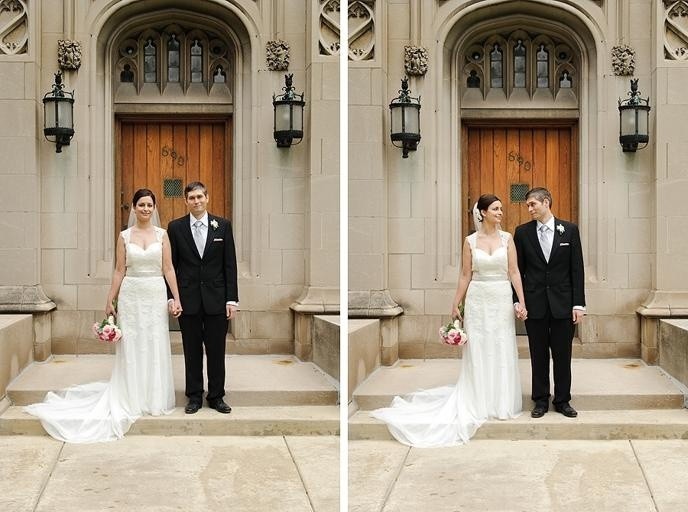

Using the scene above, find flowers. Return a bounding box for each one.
[93,298,122,342]
[439,305,467,347]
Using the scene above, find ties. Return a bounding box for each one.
[193,221,205,257]
[539,225,550,263]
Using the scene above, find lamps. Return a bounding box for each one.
[389,75,422,158]
[42,71,75,153]
[272,73,305,148]
[618,78,652,152]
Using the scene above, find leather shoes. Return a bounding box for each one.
[185,401,202,414]
[531,407,548,417]
[208,398,231,413]
[555,403,577,416]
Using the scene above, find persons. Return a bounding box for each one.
[163,181,239,415]
[22,189,183,445]
[511,187,586,418]
[368,193,529,449]
